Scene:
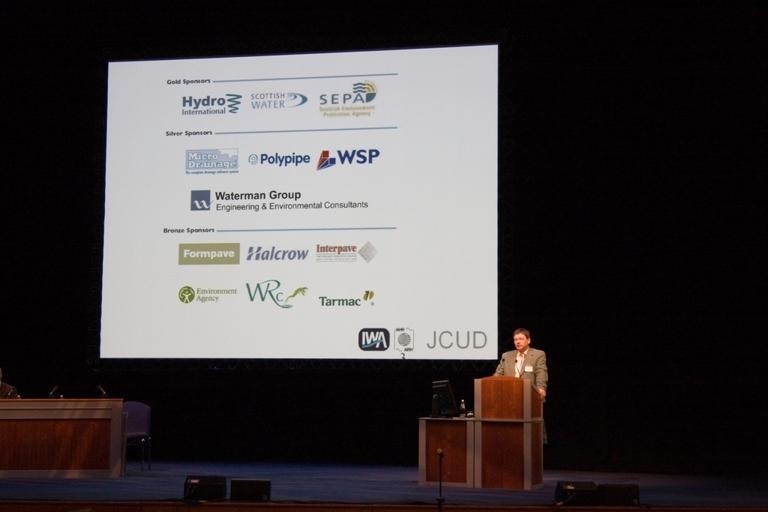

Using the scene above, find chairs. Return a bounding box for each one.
[122,401,152,473]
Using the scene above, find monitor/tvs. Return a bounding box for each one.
[432,380,460,418]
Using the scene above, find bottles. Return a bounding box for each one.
[460,399,466,417]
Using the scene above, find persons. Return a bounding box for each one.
[495,328,549,448]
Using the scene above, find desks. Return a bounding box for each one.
[0,398,128,480]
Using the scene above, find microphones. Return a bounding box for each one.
[49,386,58,396]
[493,359,505,376]
[515,360,521,376]
[7,386,15,396]
[98,384,107,396]
